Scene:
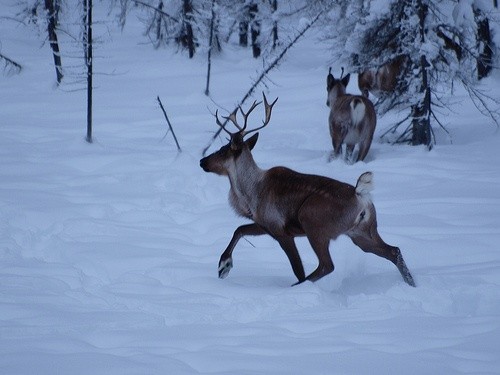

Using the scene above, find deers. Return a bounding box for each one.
[357,53,412,107]
[199,90,417,288]
[325,66,378,163]
